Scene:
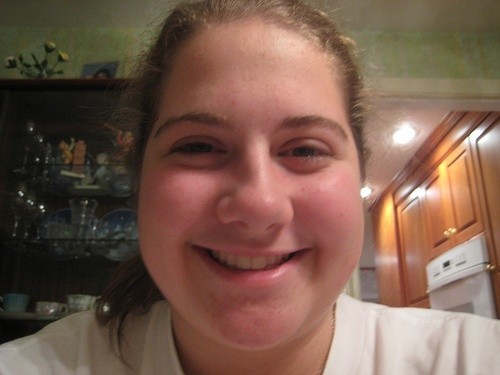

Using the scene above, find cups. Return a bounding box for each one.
[69,197,98,238]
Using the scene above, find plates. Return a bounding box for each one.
[99,209,140,261]
[37,208,75,259]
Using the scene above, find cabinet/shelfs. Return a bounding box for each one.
[0,77,153,341]
[367,112,500,321]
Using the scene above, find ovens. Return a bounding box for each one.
[425,232,496,319]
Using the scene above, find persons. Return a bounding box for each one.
[0,0,500,375]
[63,137,75,164]
[115,128,135,159]
[91,152,110,185]
[86,67,112,80]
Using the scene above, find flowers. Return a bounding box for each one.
[3,39,70,79]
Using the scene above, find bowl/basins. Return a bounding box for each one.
[35,301,68,315]
[0,293,30,313]
[66,294,102,312]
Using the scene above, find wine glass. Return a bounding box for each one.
[8,189,49,238]
[20,121,35,164]
